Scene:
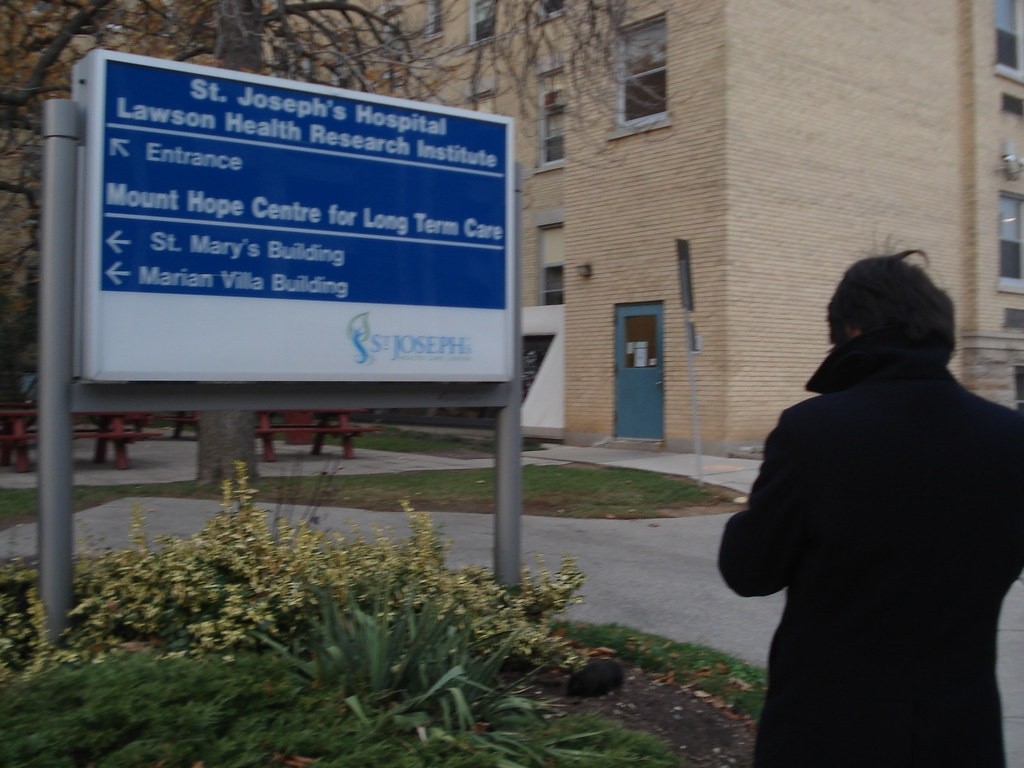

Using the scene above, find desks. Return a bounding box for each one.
[0,409,138,473]
[253,409,371,462]
[0,402,37,407]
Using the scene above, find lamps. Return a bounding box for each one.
[574,262,592,278]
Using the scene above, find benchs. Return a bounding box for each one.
[0,429,164,442]
[252,424,386,435]
[126,415,198,422]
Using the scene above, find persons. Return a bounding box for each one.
[719,231,1024,767]
